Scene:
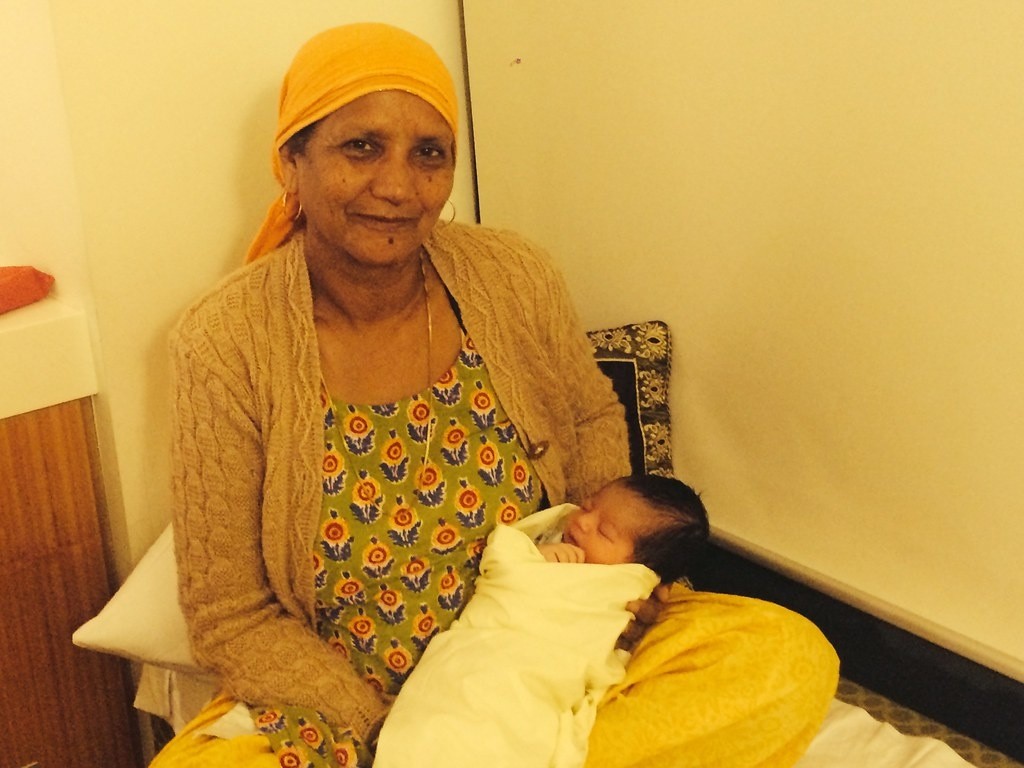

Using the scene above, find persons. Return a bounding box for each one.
[148,22,844,768]
[370,473,712,768]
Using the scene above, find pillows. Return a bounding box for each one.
[71,518,225,688]
[586,322,671,480]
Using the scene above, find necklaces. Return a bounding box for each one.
[324,247,433,513]
